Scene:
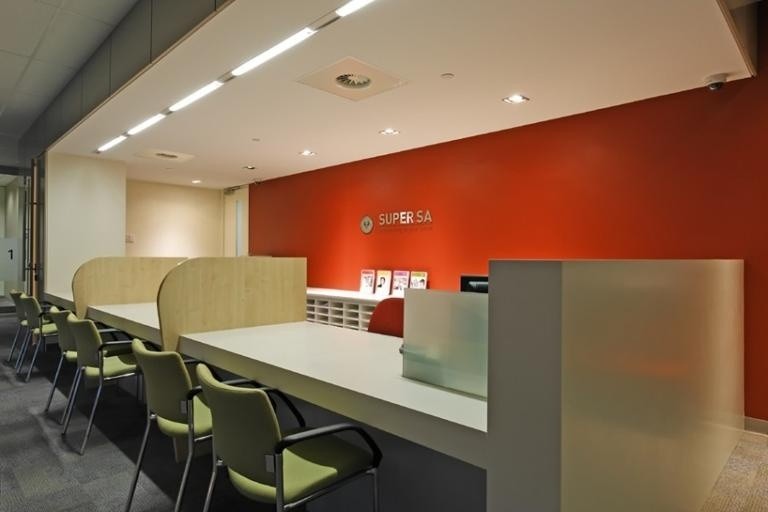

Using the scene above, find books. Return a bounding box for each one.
[360,269,375,293]
[410,271,428,289]
[392,270,410,296]
[375,270,392,295]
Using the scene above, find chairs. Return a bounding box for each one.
[367,296,406,337]
[194,362,383,511]
[4,286,161,456]
[125,337,263,512]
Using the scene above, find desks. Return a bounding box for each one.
[178,318,488,467]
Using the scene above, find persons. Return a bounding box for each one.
[378,277,385,287]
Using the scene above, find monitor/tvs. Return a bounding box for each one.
[460,274,488,293]
